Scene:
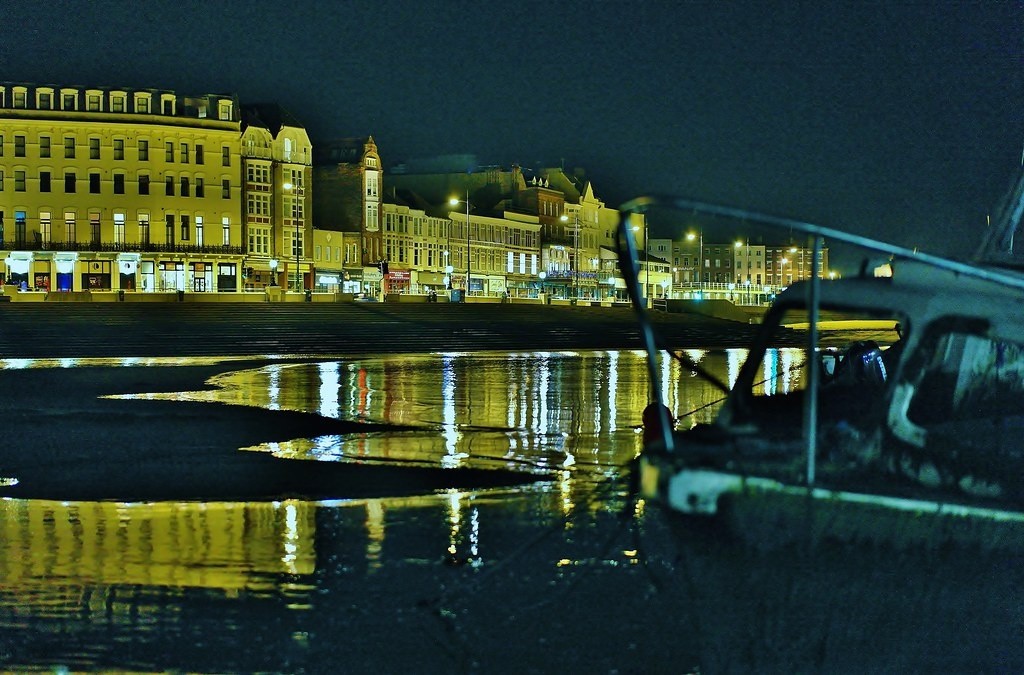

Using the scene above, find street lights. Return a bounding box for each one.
[560,213,579,299]
[631,217,649,296]
[735,237,750,305]
[688,226,703,299]
[284,177,299,292]
[790,243,805,281]
[450,189,471,295]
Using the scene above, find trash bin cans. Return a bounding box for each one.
[305,290,312,302]
[429,291,437,302]
[451,288,465,303]
[501,295,507,303]
[119,291,124,302]
[179,291,184,301]
[571,298,578,305]
